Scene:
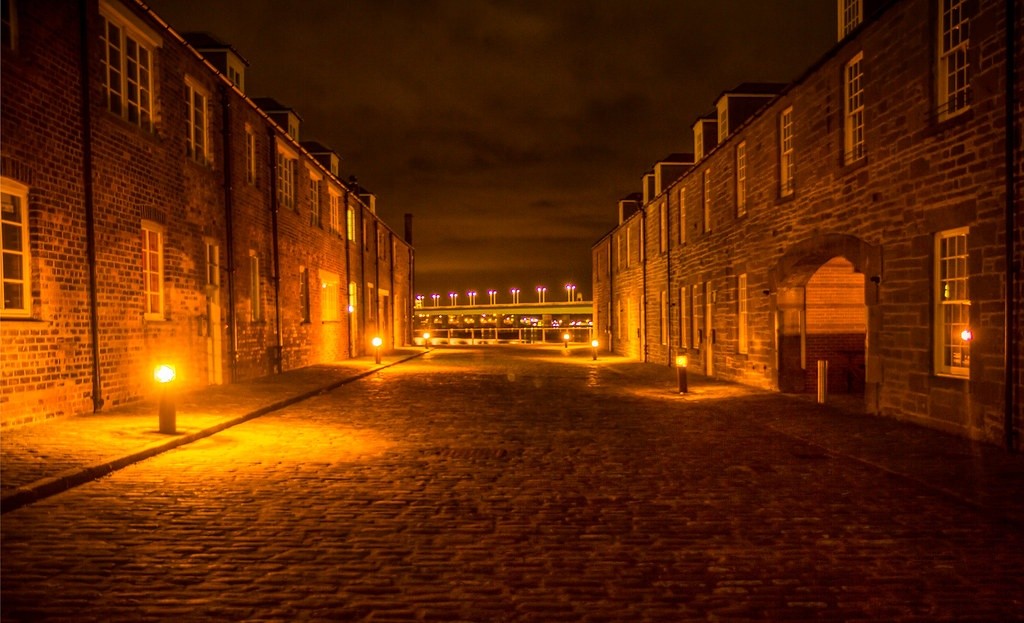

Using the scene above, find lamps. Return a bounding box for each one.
[154,354,178,434]
[374,336,382,364]
[564,333,569,348]
[675,355,689,393]
[592,338,598,360]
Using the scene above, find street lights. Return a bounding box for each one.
[511,288,520,304]
[488,289,498,305]
[416,294,425,307]
[468,291,476,306]
[537,286,546,303]
[449,293,458,306]
[432,294,440,307]
[566,284,576,302]
